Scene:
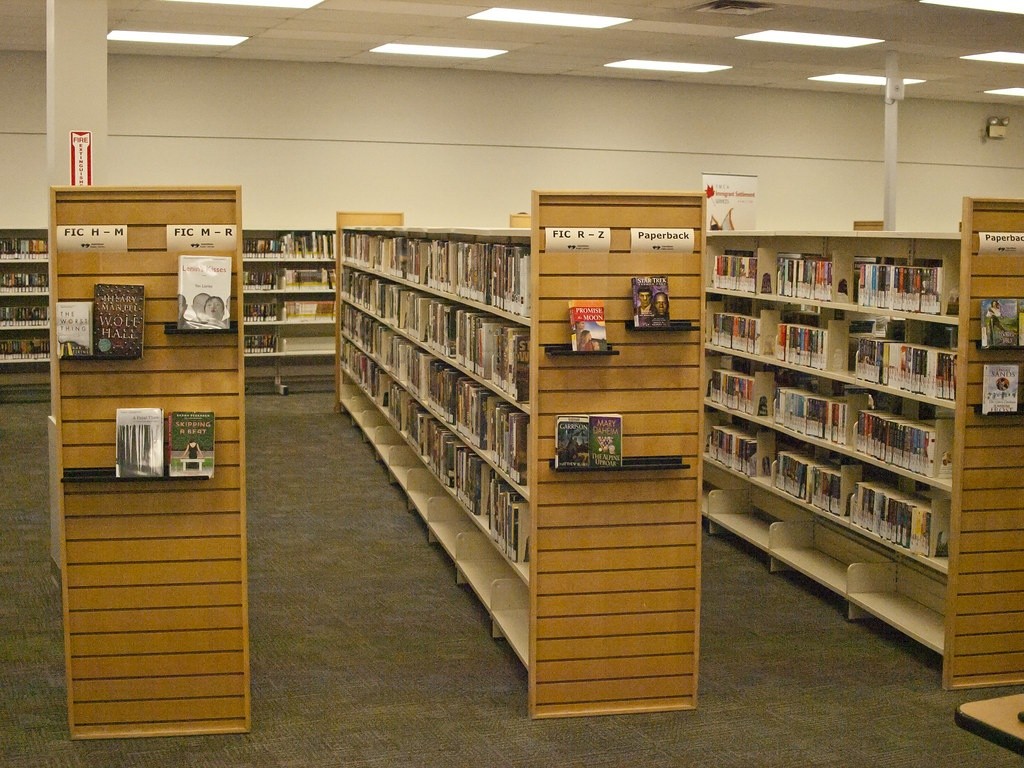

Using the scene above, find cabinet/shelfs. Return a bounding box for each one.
[0,227,50,364]
[48,185,252,742]
[510,195,1024,692]
[334,189,706,721]
[242,228,336,396]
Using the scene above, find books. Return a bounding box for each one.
[178,255,232,330]
[168,412,216,477]
[630,274,672,329]
[91,283,147,358]
[0,237,50,361]
[707,256,1024,559]
[56,300,92,357]
[117,406,165,476]
[244,231,531,564]
[567,299,610,352]
[554,411,622,469]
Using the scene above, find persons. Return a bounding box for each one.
[576,321,598,350]
[638,283,668,315]
[984,301,1007,347]
[179,292,229,329]
[182,440,203,458]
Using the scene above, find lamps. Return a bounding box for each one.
[986,117,1010,138]
